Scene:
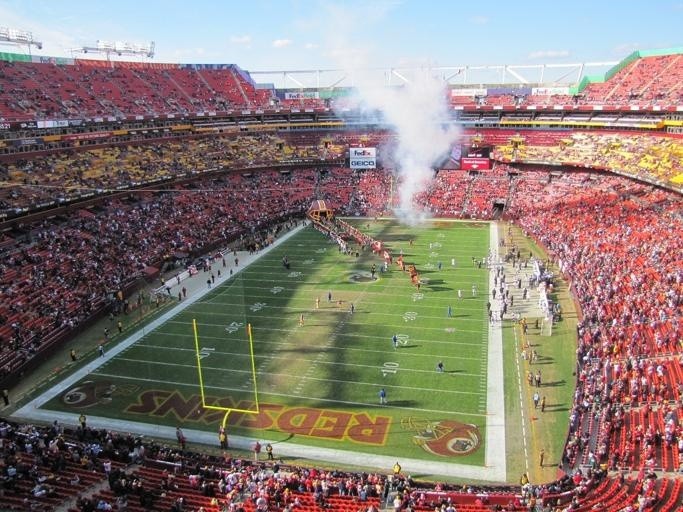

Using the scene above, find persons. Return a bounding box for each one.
[0,53,682,512]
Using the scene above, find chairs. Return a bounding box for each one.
[0,55,683,512]
